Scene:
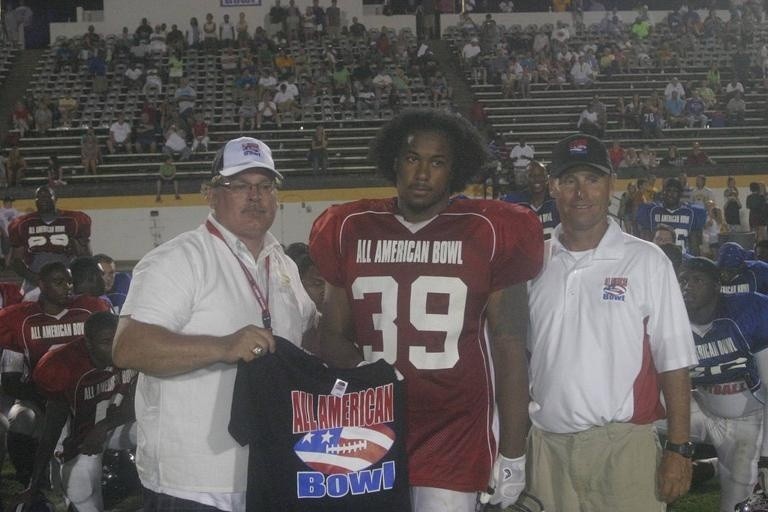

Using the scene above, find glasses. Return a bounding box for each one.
[220,180,277,194]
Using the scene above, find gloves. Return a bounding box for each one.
[480,453,527,509]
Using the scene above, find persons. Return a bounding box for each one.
[1,1,768,512]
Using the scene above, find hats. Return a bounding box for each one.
[550,133,613,178]
[211,136,284,181]
[718,242,745,268]
